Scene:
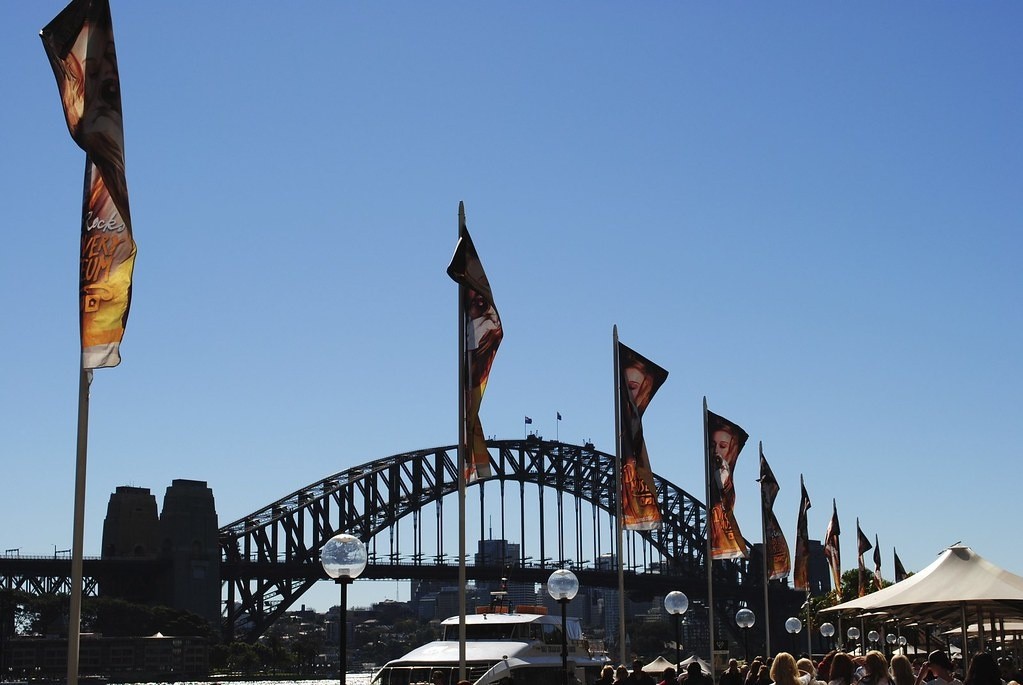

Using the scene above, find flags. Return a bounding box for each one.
[873,534,882,591]
[707,410,750,560]
[756,454,791,580]
[38,0,137,380]
[618,342,668,530]
[557,412,561,420]
[447,226,504,486]
[525,417,532,424]
[894,548,908,583]
[793,484,812,591]
[824,505,841,601]
[856,519,872,598]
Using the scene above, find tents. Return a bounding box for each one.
[818,542,1023,674]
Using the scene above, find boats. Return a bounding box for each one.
[371,576,612,685]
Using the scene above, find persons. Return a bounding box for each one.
[684,662,714,685]
[623,358,653,408]
[625,659,656,685]
[596,665,615,685]
[721,650,1023,685]
[710,423,739,494]
[660,667,685,685]
[613,665,629,685]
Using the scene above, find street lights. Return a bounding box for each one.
[886,634,897,659]
[664,591,689,677]
[735,609,756,665]
[785,617,802,659]
[820,623,835,653]
[847,627,860,657]
[867,631,880,650]
[897,636,907,656]
[547,568,579,685]
[320,534,368,685]
